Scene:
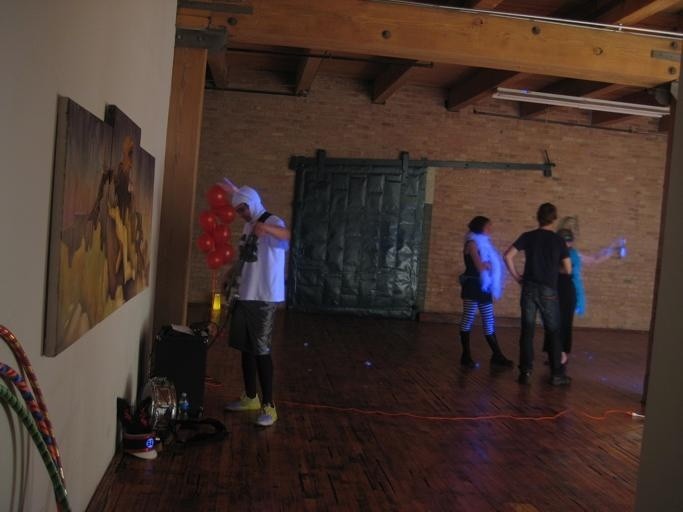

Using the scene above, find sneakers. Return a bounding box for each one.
[518,370,532,385]
[551,375,571,386]
[253,400,278,426]
[223,391,262,412]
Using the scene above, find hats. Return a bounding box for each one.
[233,185,265,218]
[122,420,155,452]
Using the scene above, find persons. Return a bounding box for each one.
[502,202,574,388]
[113,136,135,227]
[542,227,627,371]
[220,184,291,428]
[459,215,512,370]
[88,168,126,308]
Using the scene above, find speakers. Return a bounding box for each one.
[148,323,208,422]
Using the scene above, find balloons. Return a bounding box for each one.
[198,186,236,270]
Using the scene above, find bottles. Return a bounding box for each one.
[176,393,188,418]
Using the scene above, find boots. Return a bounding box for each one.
[459,330,476,367]
[485,334,512,363]
